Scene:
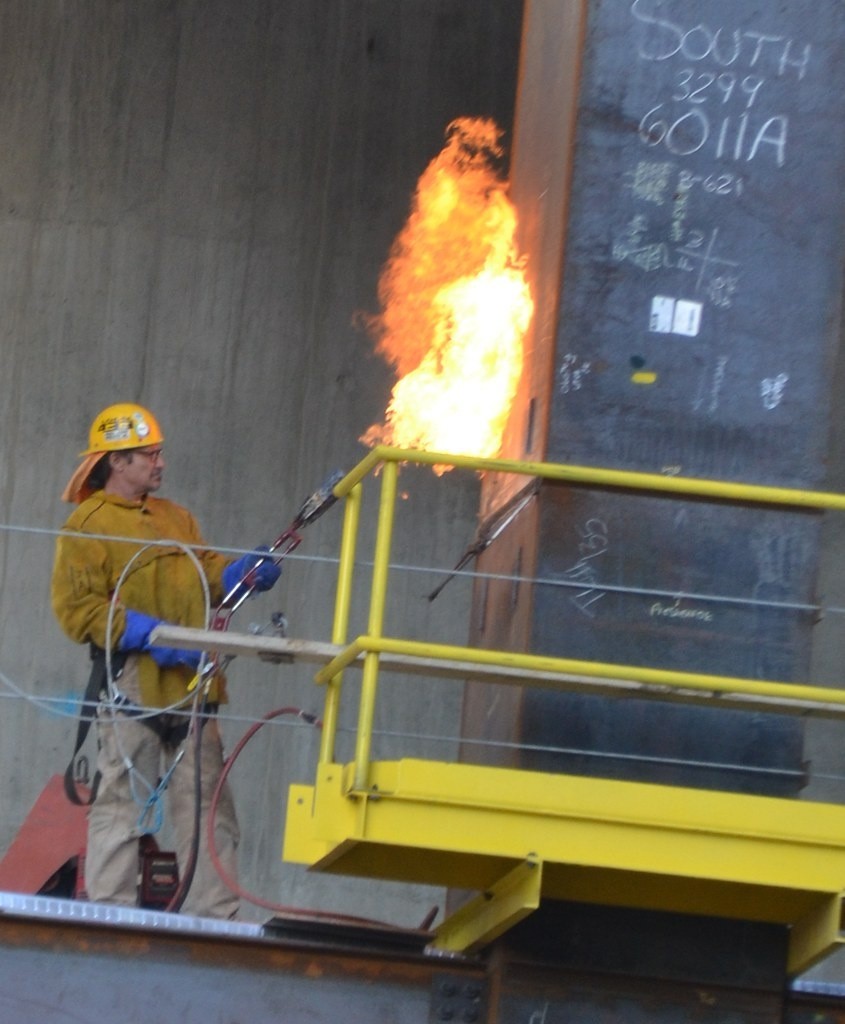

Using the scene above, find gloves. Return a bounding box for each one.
[119,607,210,672]
[221,543,282,601]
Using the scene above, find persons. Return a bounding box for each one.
[53,403,281,924]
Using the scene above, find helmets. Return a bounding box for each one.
[77,402,164,456]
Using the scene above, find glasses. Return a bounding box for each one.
[124,448,163,462]
